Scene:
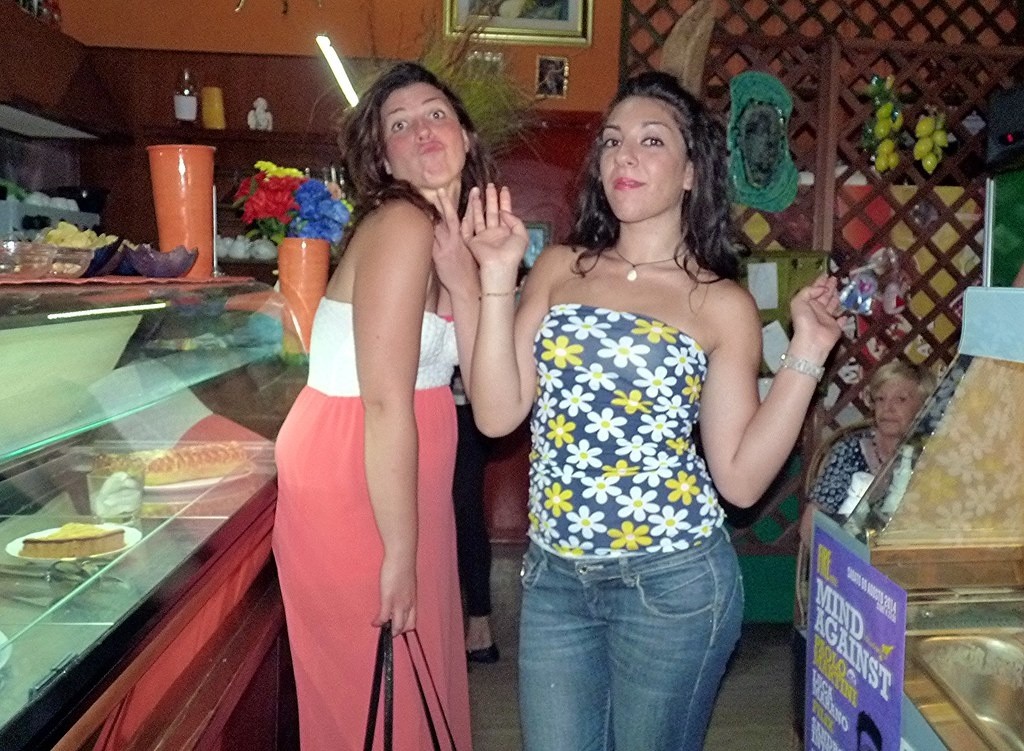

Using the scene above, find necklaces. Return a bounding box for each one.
[613,237,689,283]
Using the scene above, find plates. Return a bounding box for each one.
[5,523,142,568]
[142,460,256,494]
[0,631,12,669]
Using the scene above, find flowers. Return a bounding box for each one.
[232,159,355,246]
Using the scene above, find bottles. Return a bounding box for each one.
[173,68,198,125]
[201,87,225,129]
[0,185,14,239]
[217,236,277,260]
[145,145,212,278]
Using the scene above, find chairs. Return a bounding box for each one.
[796,418,878,631]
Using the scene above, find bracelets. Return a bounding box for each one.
[478,285,521,299]
[778,353,826,384]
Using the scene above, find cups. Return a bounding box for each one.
[86,466,145,517]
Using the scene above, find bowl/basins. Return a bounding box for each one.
[123,242,198,277]
[57,185,109,211]
[25,191,80,211]
[79,237,124,278]
[0,240,57,279]
[42,246,94,279]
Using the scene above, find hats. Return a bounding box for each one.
[726,70,799,213]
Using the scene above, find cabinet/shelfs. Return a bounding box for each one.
[143,124,341,290]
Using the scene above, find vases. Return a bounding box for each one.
[277,237,331,355]
[146,143,217,277]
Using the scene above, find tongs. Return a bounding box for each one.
[0,558,121,582]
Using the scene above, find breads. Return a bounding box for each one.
[19,522,124,560]
[91,440,250,484]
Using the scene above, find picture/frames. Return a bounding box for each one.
[442,0,594,48]
[534,55,570,101]
[468,50,504,77]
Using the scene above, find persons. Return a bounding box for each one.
[798,361,936,556]
[448,355,500,674]
[460,70,849,751]
[34,381,206,605]
[268,61,487,751]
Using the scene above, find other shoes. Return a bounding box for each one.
[465,644,500,663]
